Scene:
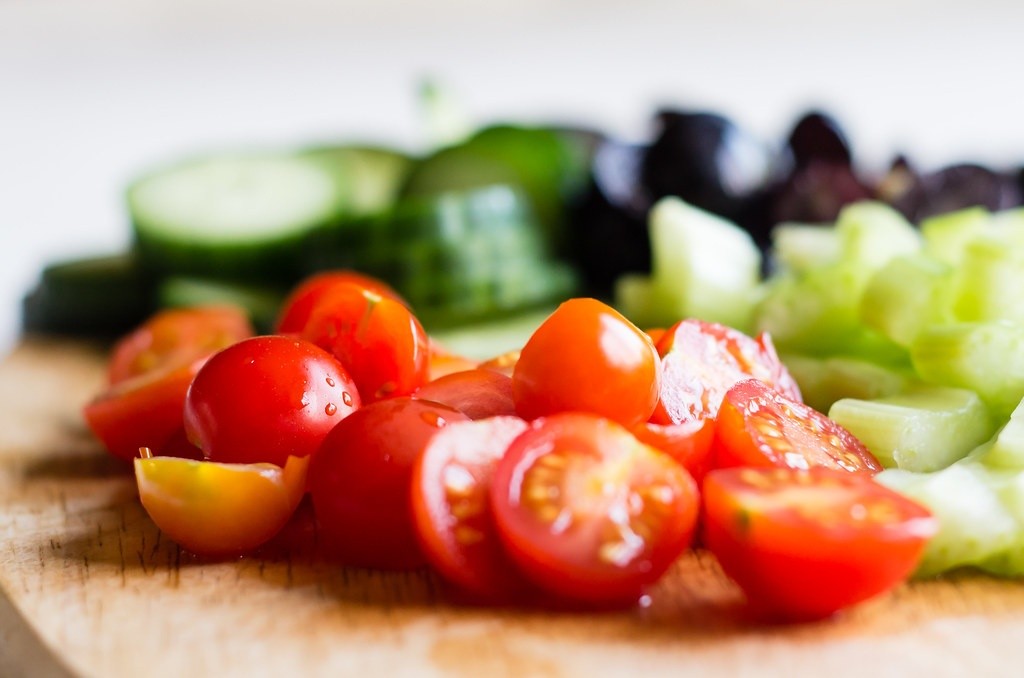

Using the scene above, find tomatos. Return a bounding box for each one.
[79,270,936,618]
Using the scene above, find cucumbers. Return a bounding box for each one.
[15,122,600,334]
